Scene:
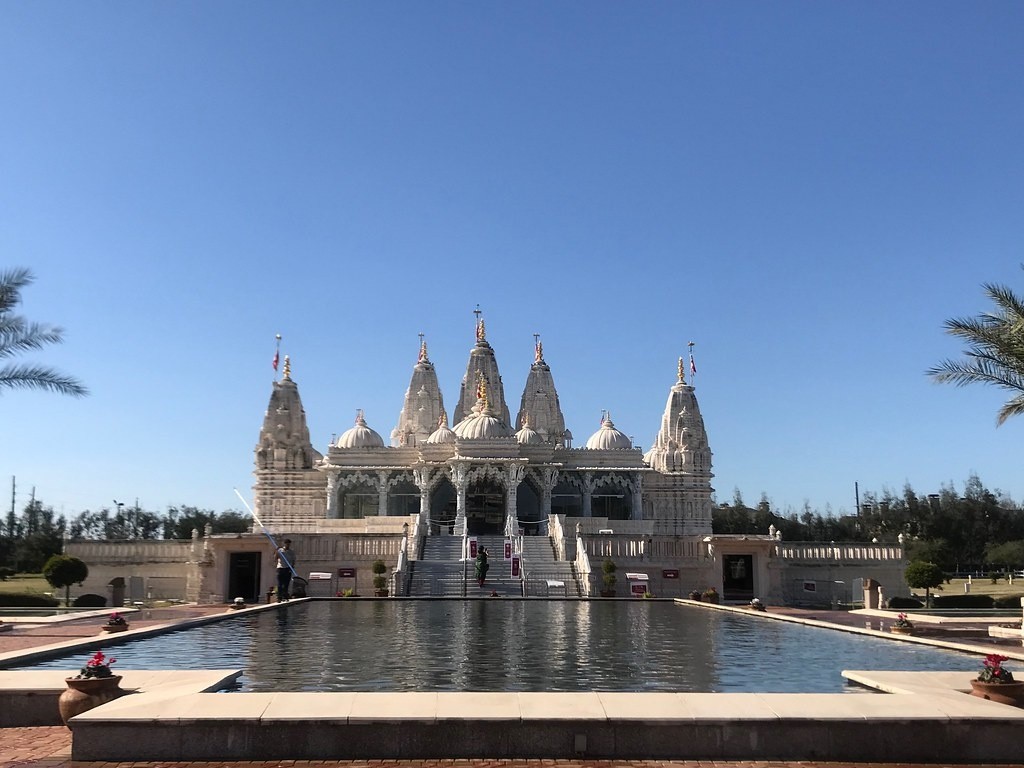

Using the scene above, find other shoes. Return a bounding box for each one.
[278,597,282,603]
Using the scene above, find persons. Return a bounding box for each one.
[274,539,296,603]
[473,545,489,588]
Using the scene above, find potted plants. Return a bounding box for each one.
[600,557,619,597]
[372,559,389,597]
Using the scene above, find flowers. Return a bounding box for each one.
[106,612,126,625]
[234,597,245,605]
[689,589,700,595]
[707,586,720,599]
[74,650,117,679]
[702,590,708,599]
[894,611,912,627]
[977,654,1016,685]
[336,592,358,596]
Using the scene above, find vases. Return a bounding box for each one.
[689,595,701,601]
[99,624,130,636]
[889,625,918,636]
[702,598,707,602]
[707,597,719,604]
[225,604,247,613]
[59,675,123,742]
[969,679,1024,709]
